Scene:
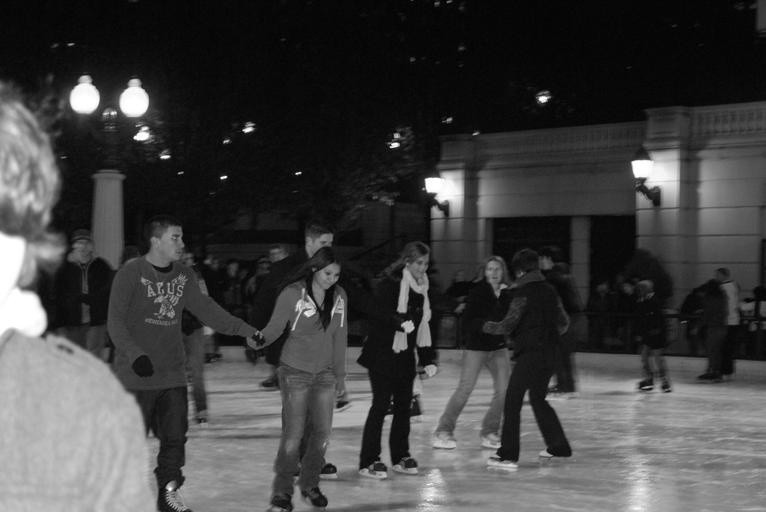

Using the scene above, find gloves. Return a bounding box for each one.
[253,331,265,346]
[132,355,153,377]
[401,320,415,334]
[424,364,438,378]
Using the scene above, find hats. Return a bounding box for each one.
[71,229,94,241]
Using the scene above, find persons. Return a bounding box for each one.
[59,228,115,357]
[636,280,672,393]
[754,285,766,360]
[202,252,223,362]
[480,247,571,470]
[356,240,437,480]
[273,219,337,478]
[716,266,740,381]
[431,254,513,450]
[597,278,626,354]
[246,246,349,512]
[695,278,726,381]
[179,249,209,423]
[443,268,474,349]
[1,79,157,512]
[268,245,280,263]
[107,216,263,512]
[622,278,643,354]
[259,239,294,390]
[226,258,239,276]
[538,245,577,394]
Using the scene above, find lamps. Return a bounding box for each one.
[631,145,662,206]
[117,76,151,120]
[421,168,450,219]
[63,72,102,120]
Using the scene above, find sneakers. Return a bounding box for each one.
[300,486,328,507]
[663,383,670,389]
[484,431,502,443]
[547,385,574,393]
[368,459,386,473]
[641,380,652,386]
[434,431,455,439]
[320,463,337,474]
[409,403,421,416]
[698,373,714,380]
[487,457,518,469]
[387,402,393,412]
[333,400,348,407]
[158,490,191,511]
[539,449,553,458]
[260,377,278,387]
[398,455,417,469]
[195,409,207,422]
[272,492,294,511]
